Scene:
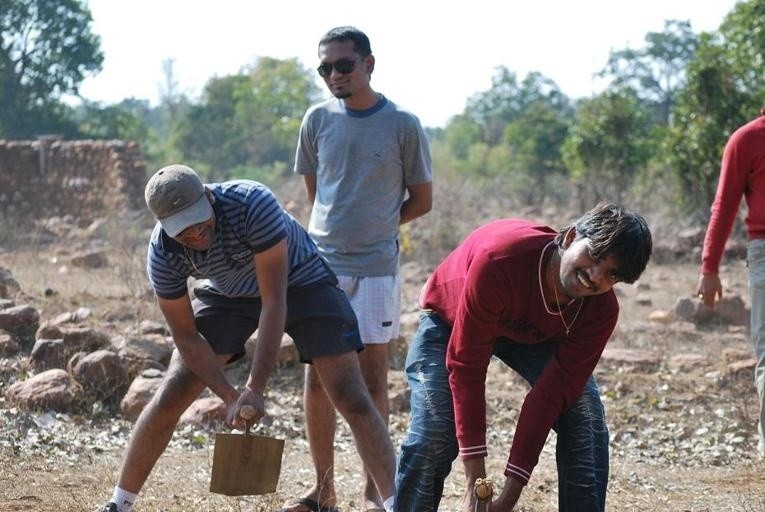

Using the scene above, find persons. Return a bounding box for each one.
[281,27,434,512]
[95,164,397,512]
[393,201,652,512]
[697,108,765,458]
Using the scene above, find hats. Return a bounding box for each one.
[144,164,213,238]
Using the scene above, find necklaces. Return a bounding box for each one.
[552,248,584,336]
[183,244,209,276]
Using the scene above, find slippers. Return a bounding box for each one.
[282,498,338,512]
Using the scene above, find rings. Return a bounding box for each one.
[699,294,703,298]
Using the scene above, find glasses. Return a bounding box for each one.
[317,55,363,78]
